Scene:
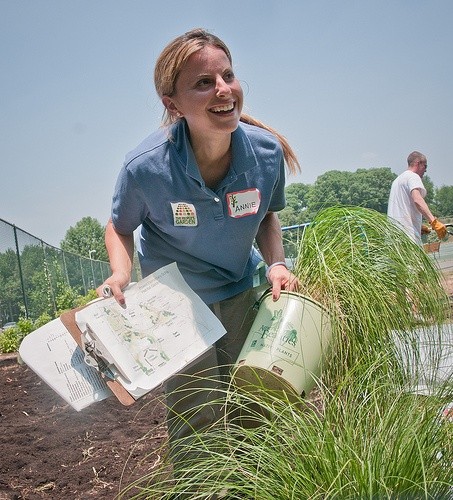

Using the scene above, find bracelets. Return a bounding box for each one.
[265,262,289,286]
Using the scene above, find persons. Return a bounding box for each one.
[387,151,447,323]
[94,29,302,500]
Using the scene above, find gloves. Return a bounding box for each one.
[421,219,448,240]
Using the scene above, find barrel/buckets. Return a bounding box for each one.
[232,291,343,410]
